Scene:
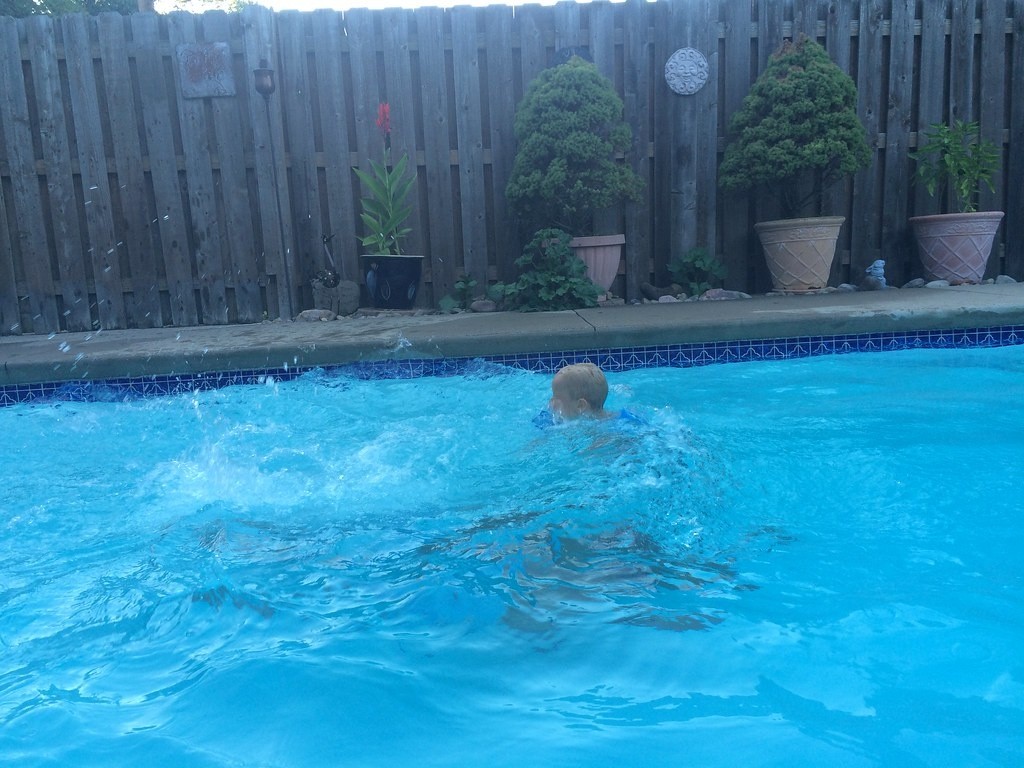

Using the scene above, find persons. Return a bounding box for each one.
[553,363,608,415]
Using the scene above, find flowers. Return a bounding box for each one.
[351,103,419,254]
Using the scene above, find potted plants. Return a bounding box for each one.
[907,118,1005,284]
[716,32,874,291]
[505,54,647,291]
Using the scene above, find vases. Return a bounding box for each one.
[359,255,425,310]
